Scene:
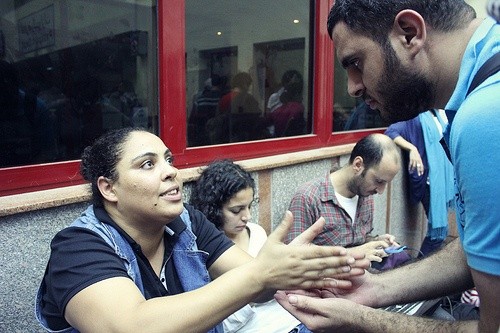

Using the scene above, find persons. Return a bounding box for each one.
[188,159,314,333]
[0,19,166,168]
[273,0,500,333]
[384,108,455,258]
[278,133,458,322]
[186,60,306,149]
[333,97,391,132]
[34,126,371,333]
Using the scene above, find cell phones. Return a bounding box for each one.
[385,246,407,253]
